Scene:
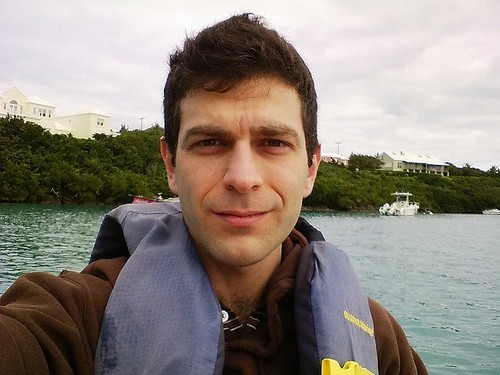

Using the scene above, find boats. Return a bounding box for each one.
[132,194,181,204]
[378,191,421,216]
[482,208,500,215]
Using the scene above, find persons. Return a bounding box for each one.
[0,13,429,374]
